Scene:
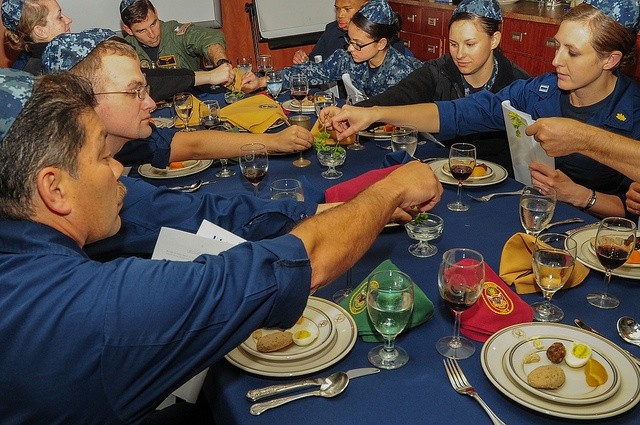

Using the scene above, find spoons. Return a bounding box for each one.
[168,179,203,194]
[617,315,640,346]
[250,371,349,416]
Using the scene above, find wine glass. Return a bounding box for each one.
[239,143,268,199]
[236,56,253,73]
[199,100,221,131]
[290,74,309,115]
[332,268,356,303]
[530,233,577,322]
[367,271,413,370]
[209,125,237,178]
[446,143,477,212]
[270,179,304,201]
[347,131,365,151]
[289,116,312,168]
[392,125,418,157]
[314,91,335,118]
[257,55,272,72]
[266,78,283,103]
[587,217,636,310]
[519,184,557,237]
[434,248,485,360]
[173,92,196,131]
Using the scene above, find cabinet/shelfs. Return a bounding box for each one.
[387,0,445,61]
[500,12,556,77]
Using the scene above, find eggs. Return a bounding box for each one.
[292,328,318,347]
[566,342,592,367]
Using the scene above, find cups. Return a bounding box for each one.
[317,147,347,180]
[225,90,244,104]
[404,213,445,258]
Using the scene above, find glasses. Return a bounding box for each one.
[94,84,151,100]
[344,33,381,51]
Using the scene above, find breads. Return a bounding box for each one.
[529,365,565,390]
[257,330,292,352]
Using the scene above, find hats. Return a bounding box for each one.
[453,0,502,20]
[359,0,397,25]
[1,0,22,32]
[584,0,640,34]
[120,0,135,15]
[42,27,117,77]
[0,68,38,140]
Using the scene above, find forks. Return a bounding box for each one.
[436,358,514,425]
[467,190,522,202]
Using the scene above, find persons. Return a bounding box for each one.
[241,0,425,101]
[0,30,412,204]
[331,0,640,216]
[118,0,230,69]
[524,114,640,215]
[0,1,238,96]
[318,0,530,166]
[0,72,445,425]
[289,0,368,62]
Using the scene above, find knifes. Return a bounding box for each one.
[246,368,380,401]
[574,319,640,362]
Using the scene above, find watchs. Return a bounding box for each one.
[583,186,596,213]
[217,58,231,65]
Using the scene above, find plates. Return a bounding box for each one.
[291,101,314,109]
[358,126,416,141]
[428,157,509,186]
[563,226,640,280]
[441,161,493,181]
[239,305,335,361]
[151,159,200,173]
[480,323,640,420]
[147,117,176,128]
[505,337,621,405]
[224,295,358,377]
[282,99,336,114]
[138,160,214,178]
